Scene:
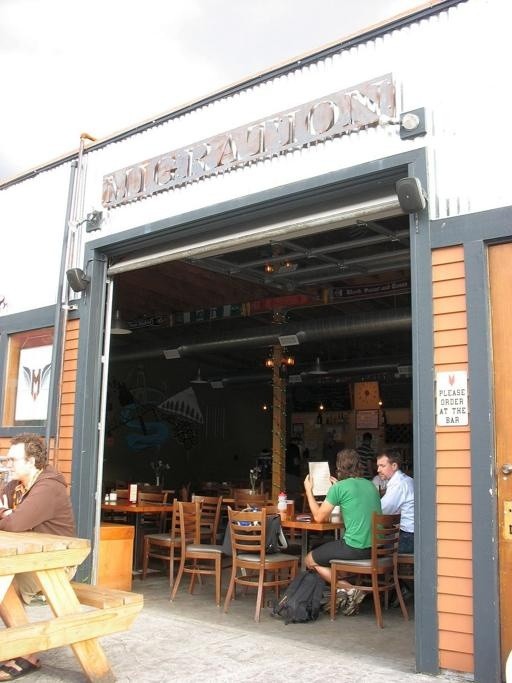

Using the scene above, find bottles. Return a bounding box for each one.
[277,490,287,510]
[105,493,109,501]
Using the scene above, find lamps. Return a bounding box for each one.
[68,210,102,234]
[109,308,135,335]
[383,104,427,144]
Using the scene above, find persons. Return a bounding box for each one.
[356,432,375,479]
[304,448,383,617]
[1,431,79,682]
[366,450,413,608]
[370,468,391,496]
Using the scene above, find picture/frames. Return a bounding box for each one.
[354,407,380,430]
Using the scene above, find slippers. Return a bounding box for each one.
[0,657,41,682]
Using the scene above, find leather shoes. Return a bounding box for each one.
[390,585,412,608]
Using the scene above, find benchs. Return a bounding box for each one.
[32,570,144,633]
[0,611,141,678]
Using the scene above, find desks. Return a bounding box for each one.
[105,501,173,580]
[278,513,345,573]
[0,522,120,683]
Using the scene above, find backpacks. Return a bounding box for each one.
[267,565,330,625]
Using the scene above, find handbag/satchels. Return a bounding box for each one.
[222,514,288,557]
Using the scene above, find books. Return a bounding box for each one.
[308,461,332,496]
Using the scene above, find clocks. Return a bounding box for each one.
[354,380,379,404]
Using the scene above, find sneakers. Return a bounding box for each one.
[341,589,368,616]
[321,590,348,615]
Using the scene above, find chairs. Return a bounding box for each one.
[384,550,415,609]
[135,491,171,568]
[223,505,298,624]
[141,497,206,573]
[169,501,225,607]
[329,509,411,628]
[104,477,389,544]
